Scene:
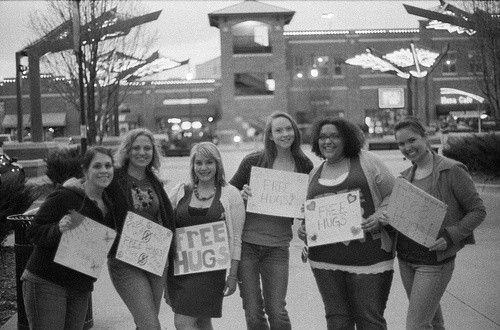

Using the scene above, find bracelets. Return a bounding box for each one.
[228,276,238,281]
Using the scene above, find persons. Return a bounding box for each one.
[162,141,246,330]
[297,114,406,330]
[380,114,487,330]
[61,126,176,330]
[223,109,314,330]
[19,143,119,330]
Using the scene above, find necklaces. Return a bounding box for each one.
[128,171,155,208]
[193,181,217,201]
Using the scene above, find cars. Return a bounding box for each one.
[0,128,221,158]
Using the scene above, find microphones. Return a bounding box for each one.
[403,157,407,160]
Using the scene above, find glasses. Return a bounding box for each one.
[318,133,342,141]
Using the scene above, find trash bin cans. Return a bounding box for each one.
[7,214,93,330]
